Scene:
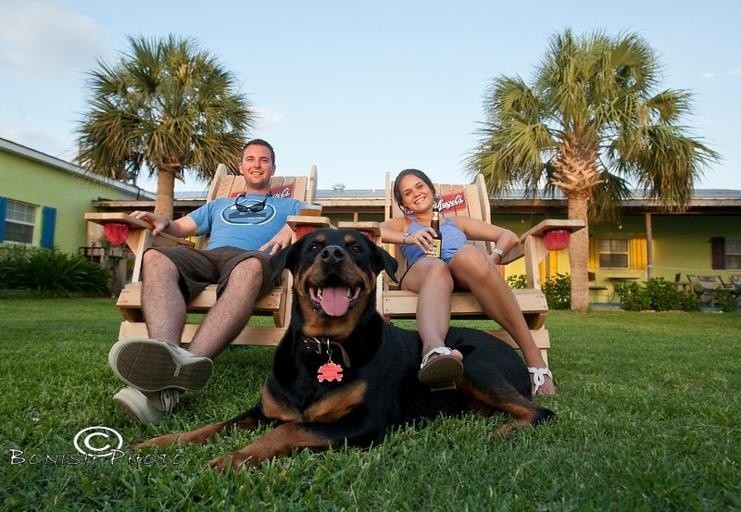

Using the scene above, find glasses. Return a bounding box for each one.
[235,194,268,212]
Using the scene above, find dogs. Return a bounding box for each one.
[126,227,558,474]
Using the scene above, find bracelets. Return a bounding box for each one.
[492,248,504,264]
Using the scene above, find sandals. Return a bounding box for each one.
[418,347,464,391]
[527,367,556,398]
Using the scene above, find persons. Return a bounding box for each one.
[108,139,310,429]
[379,169,555,396]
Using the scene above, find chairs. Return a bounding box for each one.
[84,163,338,346]
[670,273,741,307]
[588,273,609,303]
[338,172,586,368]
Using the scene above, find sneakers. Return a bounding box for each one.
[108,338,214,393]
[112,385,186,427]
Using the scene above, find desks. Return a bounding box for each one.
[607,276,640,302]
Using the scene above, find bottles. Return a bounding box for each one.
[425,207,442,258]
